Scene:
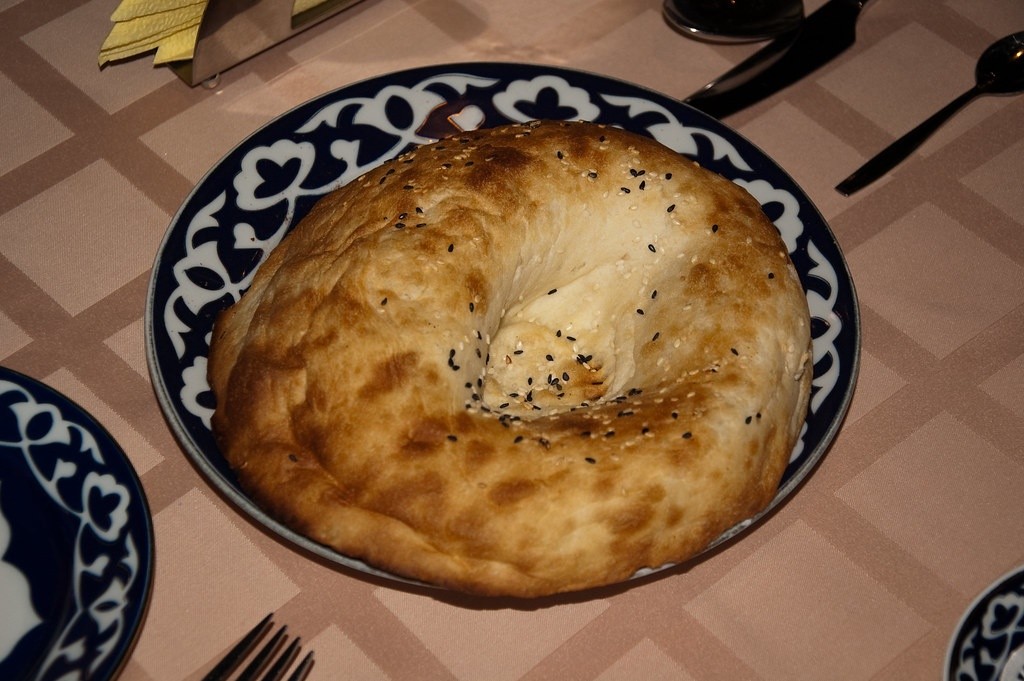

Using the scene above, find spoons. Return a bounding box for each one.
[834,31,1024,198]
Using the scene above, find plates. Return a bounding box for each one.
[0,365,158,680]
[145,60,863,591]
[941,565,1024,681]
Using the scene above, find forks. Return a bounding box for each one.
[200,612,315,681]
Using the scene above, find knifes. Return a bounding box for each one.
[681,0,869,119]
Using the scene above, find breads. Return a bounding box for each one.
[205,118,814,601]
